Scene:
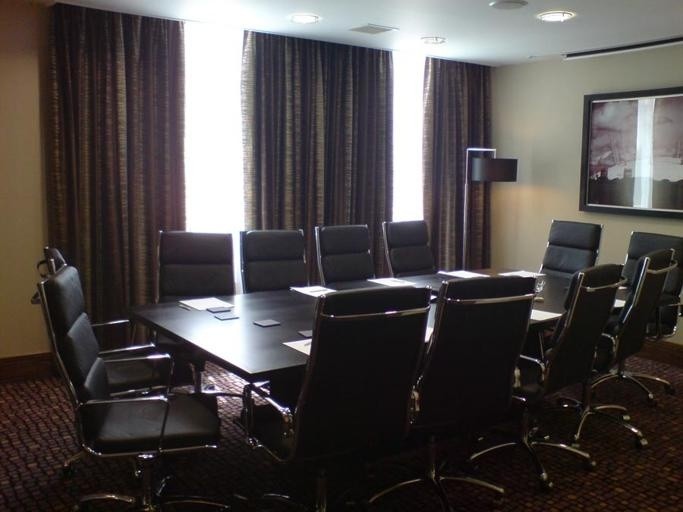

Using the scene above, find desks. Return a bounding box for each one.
[124,268,636,461]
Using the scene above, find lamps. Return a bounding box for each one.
[461,143,519,269]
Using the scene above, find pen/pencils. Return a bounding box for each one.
[310,290,327,292]
[178,304,190,310]
[304,341,311,346]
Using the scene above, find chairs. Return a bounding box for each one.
[462,258,629,491]
[26,263,237,511]
[32,239,200,481]
[577,85,682,223]
[315,220,376,285]
[379,220,439,278]
[151,227,244,403]
[589,229,681,403]
[538,218,606,282]
[229,280,436,512]
[363,273,538,512]
[557,246,679,449]
[236,223,313,295]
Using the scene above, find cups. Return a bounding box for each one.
[534,275,546,292]
